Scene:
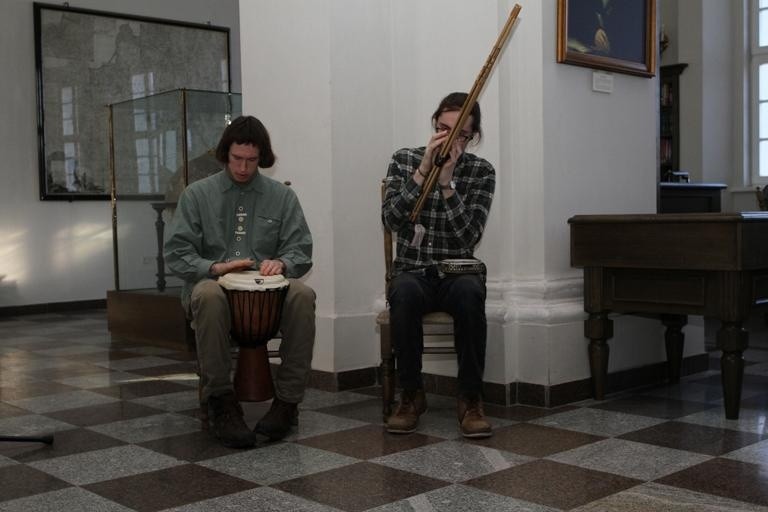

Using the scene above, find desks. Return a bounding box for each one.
[657,183,728,214]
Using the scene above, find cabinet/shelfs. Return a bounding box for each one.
[658,63,688,182]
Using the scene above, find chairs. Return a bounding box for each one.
[376,178,486,425]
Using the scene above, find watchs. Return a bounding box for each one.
[437,180,457,191]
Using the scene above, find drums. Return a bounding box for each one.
[441,260,482,274]
[217,269,290,402]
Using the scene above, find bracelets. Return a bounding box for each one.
[418,168,429,178]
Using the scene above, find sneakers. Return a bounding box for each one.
[385,385,430,434]
[210,389,256,449]
[252,394,300,445]
[454,384,494,439]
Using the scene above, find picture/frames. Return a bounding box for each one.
[557,0,656,78]
[34,3,232,202]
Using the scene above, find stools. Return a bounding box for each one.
[190,319,282,413]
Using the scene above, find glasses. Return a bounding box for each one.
[434,123,473,143]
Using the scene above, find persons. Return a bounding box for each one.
[163,114,318,449]
[380,90,498,439]
